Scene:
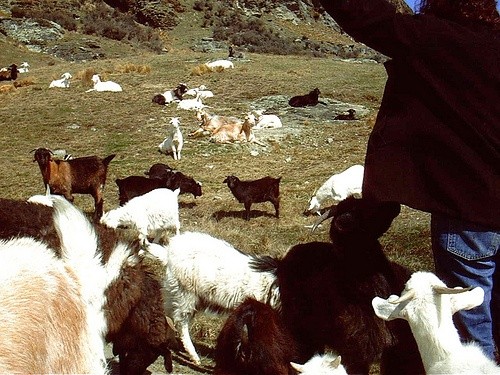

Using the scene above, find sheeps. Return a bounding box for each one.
[228,46,236,58]
[204,59,234,69]
[375,268,500,375]
[288,87,327,107]
[157,117,185,161]
[29,146,116,224]
[99,187,181,244]
[0,184,474,375]
[0,61,30,81]
[308,164,365,216]
[333,109,360,120]
[151,82,283,147]
[238,52,246,58]
[222,175,283,220]
[49,72,72,88]
[144,163,202,199]
[85,75,124,93]
[113,172,181,209]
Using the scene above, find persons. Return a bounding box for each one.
[320,0,500,359]
